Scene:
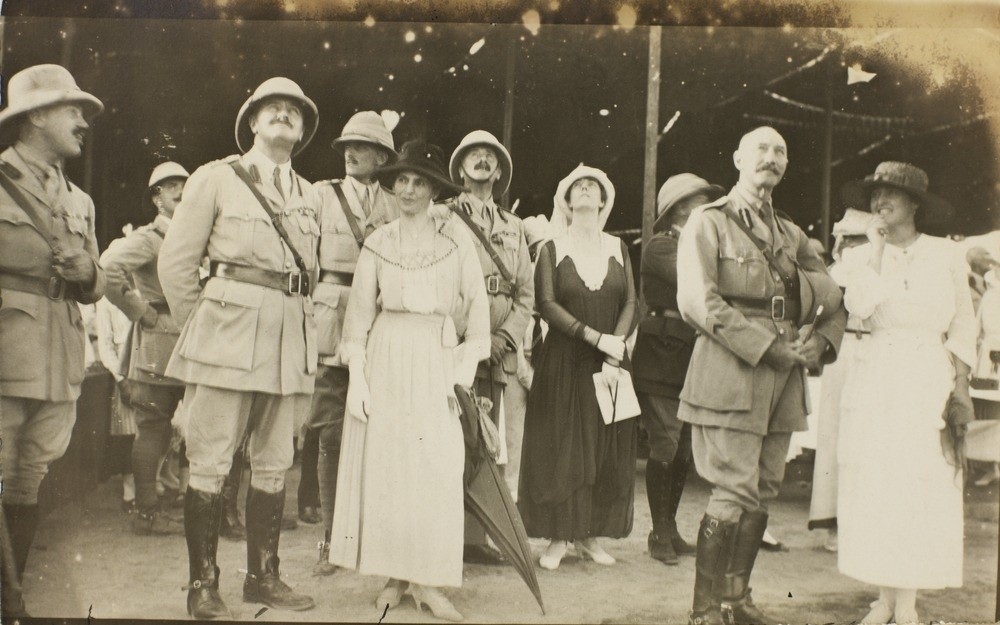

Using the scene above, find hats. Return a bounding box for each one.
[449,130,513,198]
[794,265,843,332]
[386,139,471,196]
[0,63,104,123]
[148,161,189,187]
[653,173,726,231]
[555,165,615,231]
[234,76,320,159]
[864,161,929,201]
[331,110,399,167]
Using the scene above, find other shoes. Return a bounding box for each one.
[860,598,918,625]
[157,482,168,501]
[121,493,135,511]
[761,533,788,551]
[133,508,155,533]
[314,531,339,574]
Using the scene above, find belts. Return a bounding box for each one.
[319,270,356,286]
[147,301,175,316]
[481,274,521,299]
[646,307,682,318]
[0,269,83,304]
[208,260,313,296]
[721,295,801,322]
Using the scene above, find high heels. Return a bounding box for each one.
[412,586,464,622]
[376,579,409,607]
[974,470,1000,487]
[573,538,615,566]
[539,538,568,569]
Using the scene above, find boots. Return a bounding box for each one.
[688,509,783,625]
[185,484,234,620]
[243,486,314,610]
[280,516,297,531]
[646,456,697,565]
[0,504,40,617]
[219,485,246,537]
[298,479,322,524]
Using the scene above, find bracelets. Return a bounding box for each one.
[955,371,972,382]
[819,334,833,357]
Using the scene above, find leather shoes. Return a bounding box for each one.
[464,544,508,565]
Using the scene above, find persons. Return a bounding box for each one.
[0,64,491,620]
[675,126,847,625]
[434,130,639,572]
[785,158,1000,625]
[637,172,725,569]
